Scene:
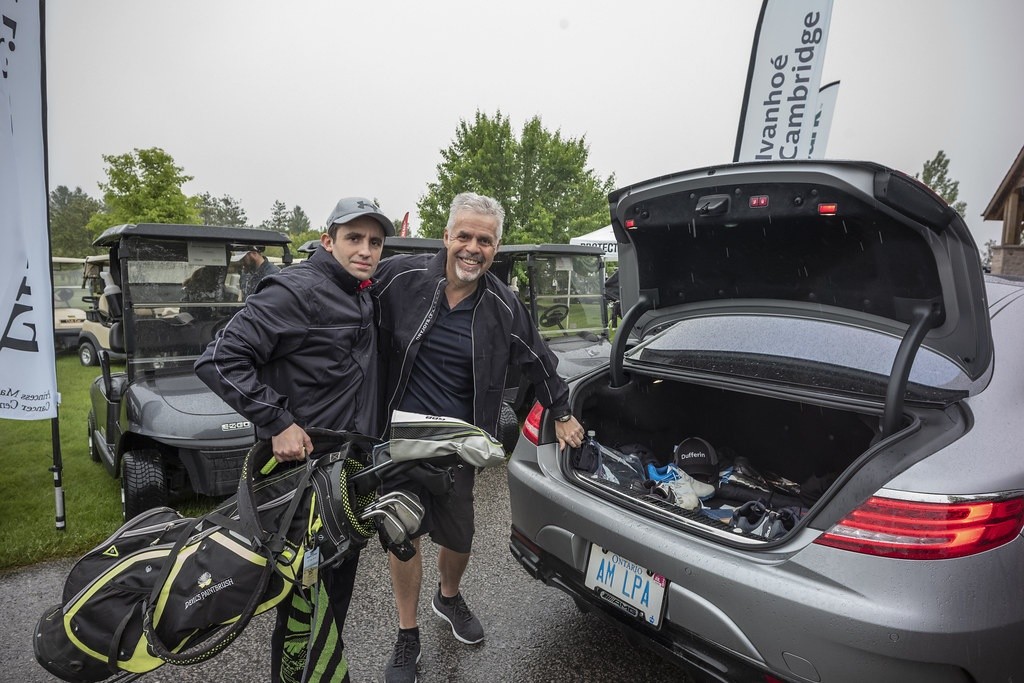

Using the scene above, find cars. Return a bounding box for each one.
[508,161,1022,683]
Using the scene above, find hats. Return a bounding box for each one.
[326,196,396,238]
[673,437,721,477]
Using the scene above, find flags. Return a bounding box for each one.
[806,74,838,159]
[735,1,835,177]
[0,0,59,419]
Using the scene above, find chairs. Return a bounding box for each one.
[99,317,190,403]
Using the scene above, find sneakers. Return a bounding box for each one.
[431,581,484,644]
[384,633,421,683]
[728,500,810,541]
[644,463,715,512]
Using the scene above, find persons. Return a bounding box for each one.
[232,247,282,306]
[332,185,589,682]
[195,193,400,681]
